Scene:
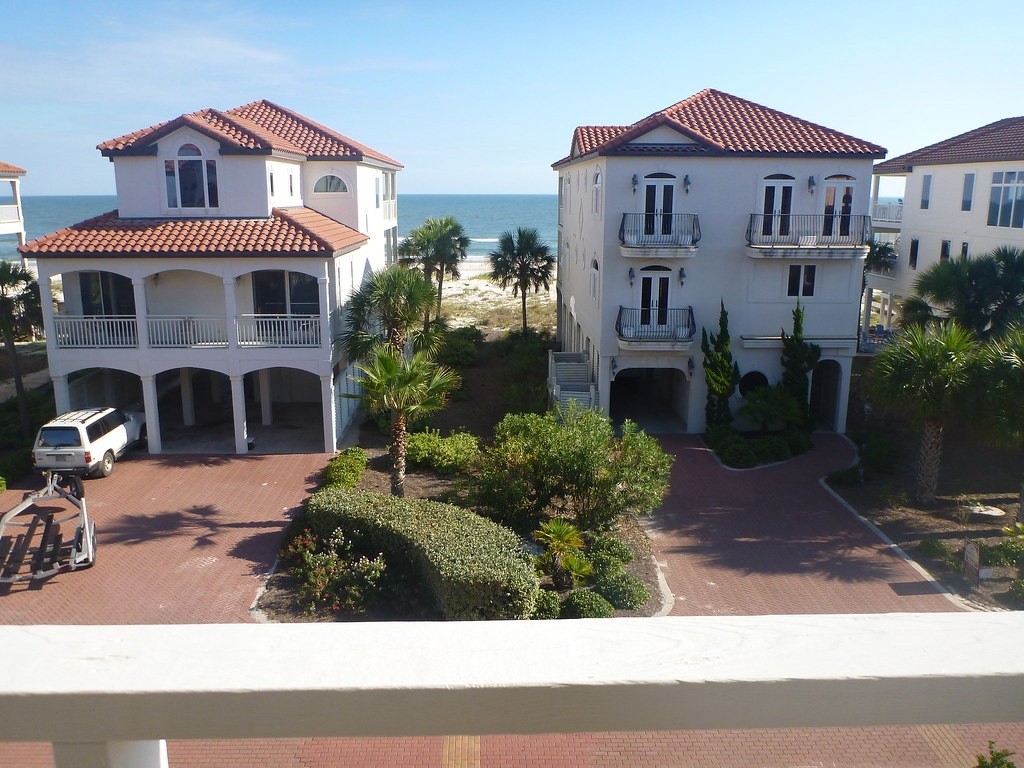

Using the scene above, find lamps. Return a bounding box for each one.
[679,266,686,288]
[153,273,159,288]
[683,175,690,195]
[688,357,694,376]
[611,357,617,377]
[808,175,815,195]
[632,173,638,195]
[629,267,635,288]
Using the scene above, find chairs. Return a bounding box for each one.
[175,316,195,341]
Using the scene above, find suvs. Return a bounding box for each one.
[32,407,147,478]
